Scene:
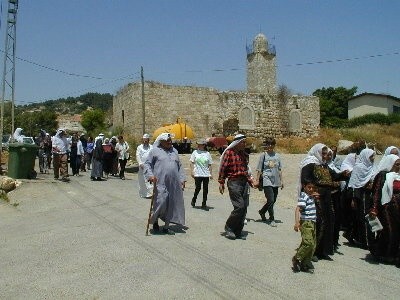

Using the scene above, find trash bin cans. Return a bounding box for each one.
[7,143,40,179]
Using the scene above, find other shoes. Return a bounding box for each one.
[225,225,236,240]
[258,210,266,221]
[269,220,277,227]
[236,231,246,240]
[191,200,196,207]
[201,202,210,212]
[292,254,333,273]
[153,221,159,233]
[163,227,175,235]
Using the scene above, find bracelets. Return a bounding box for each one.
[373,206,378,210]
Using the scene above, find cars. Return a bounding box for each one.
[2,135,39,145]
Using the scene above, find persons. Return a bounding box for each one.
[136,134,154,198]
[35,129,129,182]
[218,134,256,240]
[189,139,214,211]
[254,137,284,227]
[143,132,187,235]
[292,143,400,273]
